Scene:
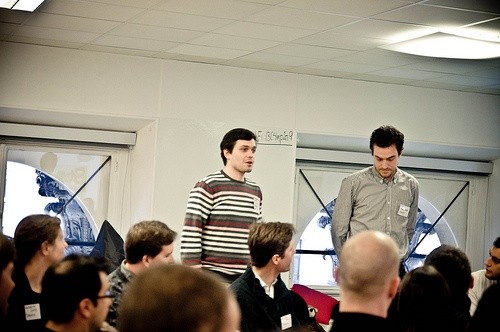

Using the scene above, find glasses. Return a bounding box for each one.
[97,290,118,301]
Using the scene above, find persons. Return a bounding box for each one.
[329,231,403,332]
[14,214,69,332]
[0,233,240,332]
[395,245,474,332]
[330,126,419,286]
[179,128,263,285]
[107,220,178,332]
[468,236,500,332]
[228,222,326,332]
